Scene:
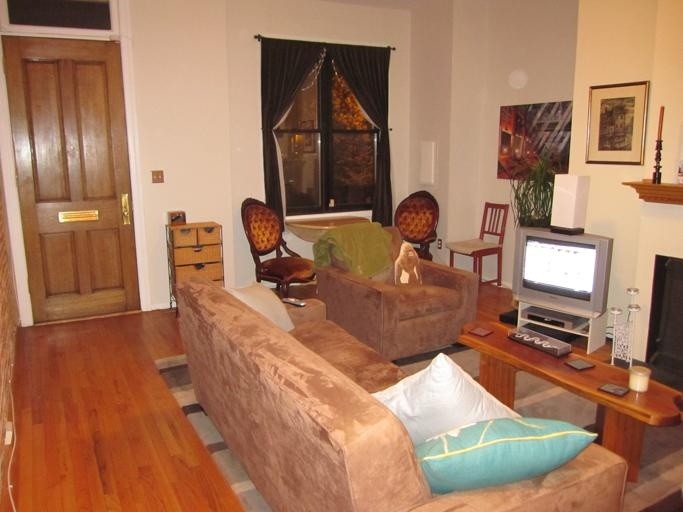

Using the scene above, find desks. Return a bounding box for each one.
[457,317,683,483]
[285,216,370,242]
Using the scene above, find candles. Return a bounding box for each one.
[657,103,665,140]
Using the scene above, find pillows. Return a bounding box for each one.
[220,281,295,332]
[394,241,423,287]
[368,353,522,447]
[412,417,599,494]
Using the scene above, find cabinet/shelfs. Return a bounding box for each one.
[165,221,226,309]
[516,302,608,354]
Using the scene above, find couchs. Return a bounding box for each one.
[172,280,628,512]
[313,222,480,361]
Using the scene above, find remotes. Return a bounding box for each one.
[282,298,307,307]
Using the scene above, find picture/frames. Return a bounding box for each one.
[584,77,651,166]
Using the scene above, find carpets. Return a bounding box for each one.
[155,348,682,512]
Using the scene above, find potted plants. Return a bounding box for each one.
[510,147,556,309]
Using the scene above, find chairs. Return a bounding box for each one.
[446,203,509,287]
[241,198,316,298]
[394,190,440,261]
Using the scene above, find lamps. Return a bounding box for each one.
[610,288,641,367]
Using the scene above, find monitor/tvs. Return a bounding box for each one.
[512,227,613,319]
